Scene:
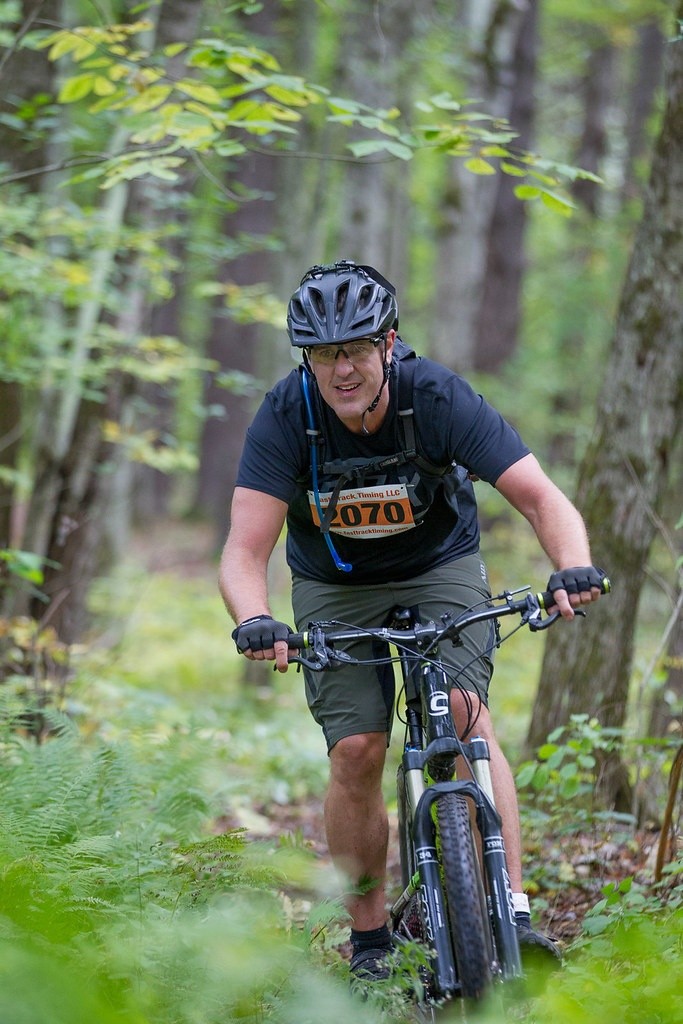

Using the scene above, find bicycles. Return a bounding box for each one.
[236,576,612,1021]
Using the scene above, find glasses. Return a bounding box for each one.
[306,330,387,365]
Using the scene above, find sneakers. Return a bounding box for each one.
[494,918,562,972]
[350,949,410,1006]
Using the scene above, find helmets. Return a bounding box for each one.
[286,260,399,348]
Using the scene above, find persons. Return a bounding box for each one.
[221,260,602,984]
